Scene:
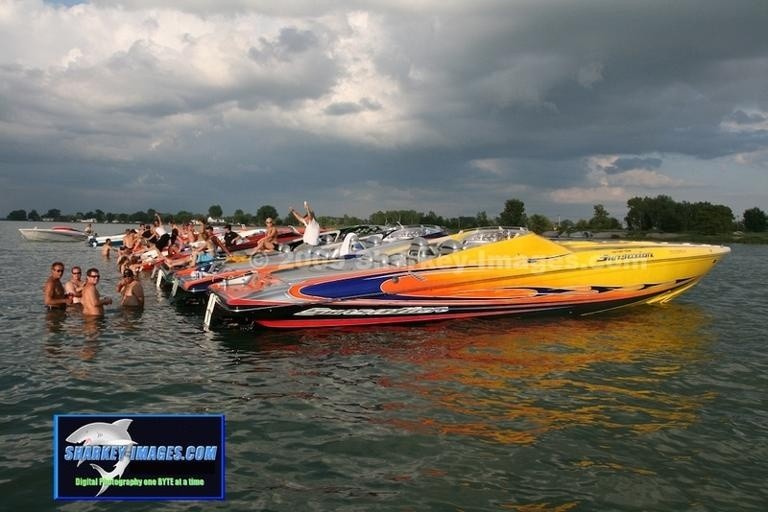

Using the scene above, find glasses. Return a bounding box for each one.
[53,268,64,273]
[88,274,100,278]
[72,271,81,275]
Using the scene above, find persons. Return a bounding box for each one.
[103,211,241,311]
[255,217,279,251]
[44,261,73,310]
[64,266,86,308]
[84,222,93,234]
[81,267,112,316]
[289,201,320,253]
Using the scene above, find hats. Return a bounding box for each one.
[123,269,134,277]
